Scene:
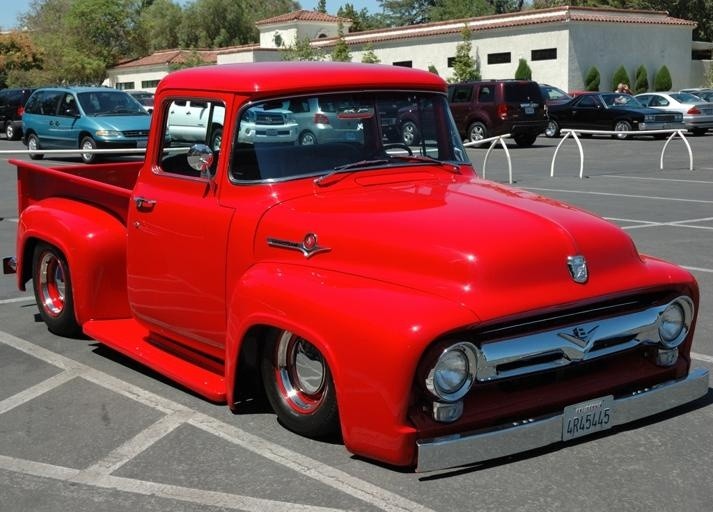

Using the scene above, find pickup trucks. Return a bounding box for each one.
[2,59,708,477]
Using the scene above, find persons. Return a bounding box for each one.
[612,82,634,104]
